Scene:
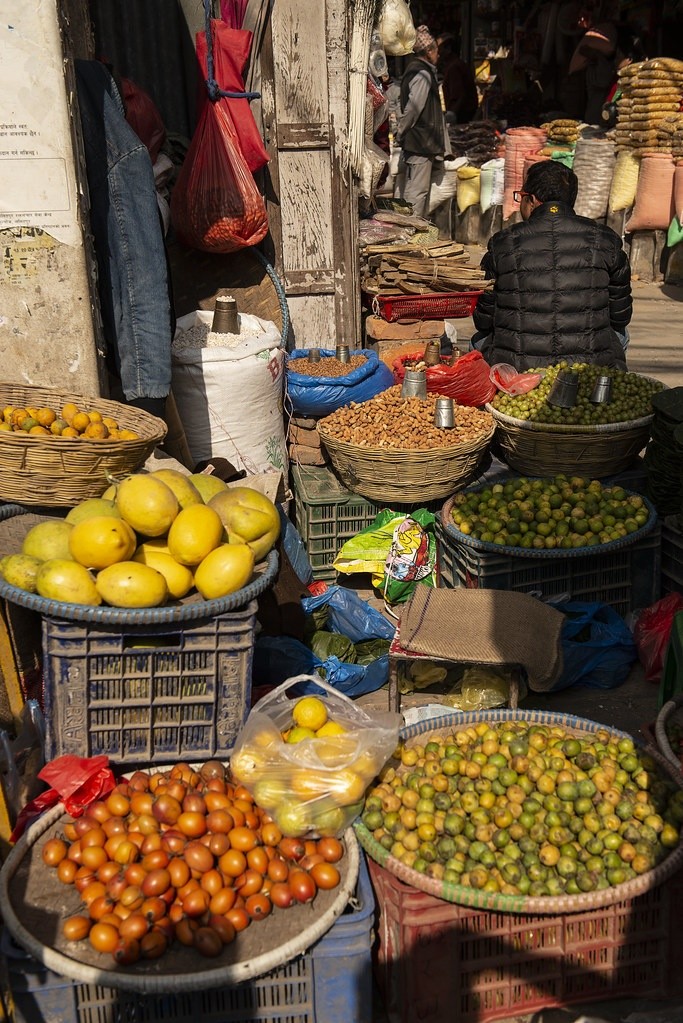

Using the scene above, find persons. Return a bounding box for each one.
[469,160,633,374]
[433,37,478,125]
[393,25,452,219]
[568,4,618,121]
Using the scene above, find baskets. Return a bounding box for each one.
[0,763,360,994]
[2,546,279,624]
[485,371,671,480]
[352,710,683,913]
[655,702,683,769]
[361,290,484,323]
[441,477,656,558]
[0,382,168,508]
[316,418,497,503]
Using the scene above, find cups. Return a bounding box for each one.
[433,398,455,428]
[211,299,240,334]
[308,349,320,363]
[545,369,580,408]
[401,370,427,400]
[423,342,440,363]
[335,344,351,363]
[450,350,463,366]
[588,375,613,406]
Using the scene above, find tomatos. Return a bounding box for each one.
[44,761,344,965]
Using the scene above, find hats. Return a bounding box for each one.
[413,25,437,56]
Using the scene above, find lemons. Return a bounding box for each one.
[451,473,649,548]
[230,696,380,839]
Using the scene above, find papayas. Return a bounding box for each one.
[0,468,281,607]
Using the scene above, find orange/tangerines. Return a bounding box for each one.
[0,402,140,440]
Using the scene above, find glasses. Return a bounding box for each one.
[513,191,534,202]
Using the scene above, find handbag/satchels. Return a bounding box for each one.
[172,100,269,253]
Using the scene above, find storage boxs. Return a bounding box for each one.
[431,519,660,618]
[0,841,376,1023]
[39,614,254,767]
[363,863,671,1023]
[289,464,382,583]
